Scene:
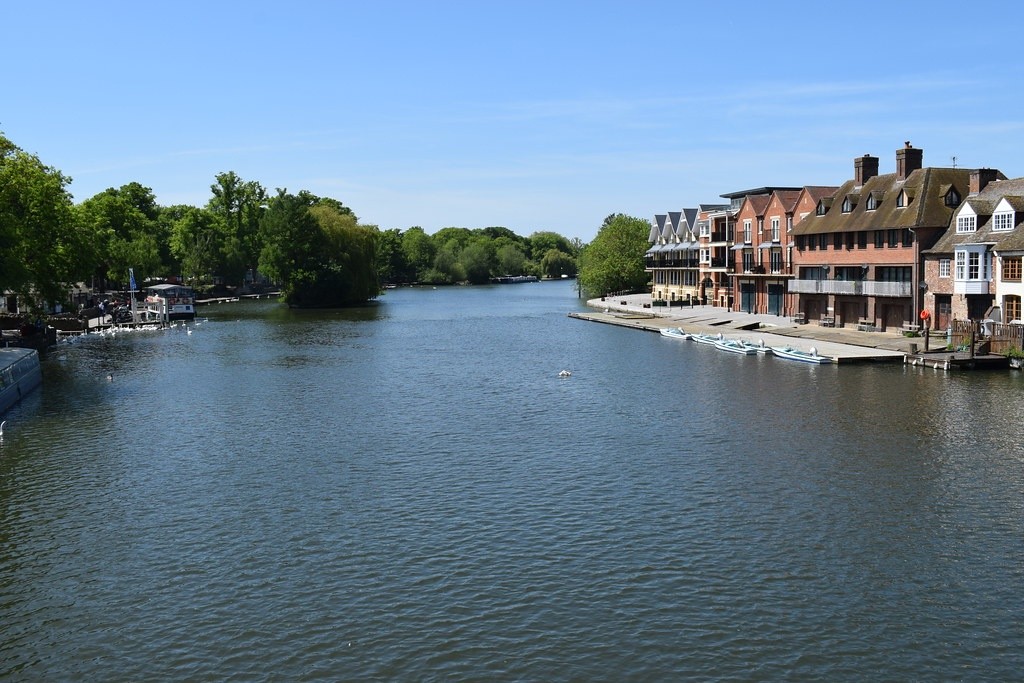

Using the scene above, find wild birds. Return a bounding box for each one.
[90,324,157,337]
[159,317,208,334]
[107,374,114,380]
[0,420,4,435]
[208,294,269,306]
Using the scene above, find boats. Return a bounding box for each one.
[690,333,727,345]
[771,347,832,364]
[714,338,757,355]
[737,340,772,354]
[497,275,537,283]
[659,327,692,340]
[147,283,195,320]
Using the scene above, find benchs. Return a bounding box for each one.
[790,312,921,335]
